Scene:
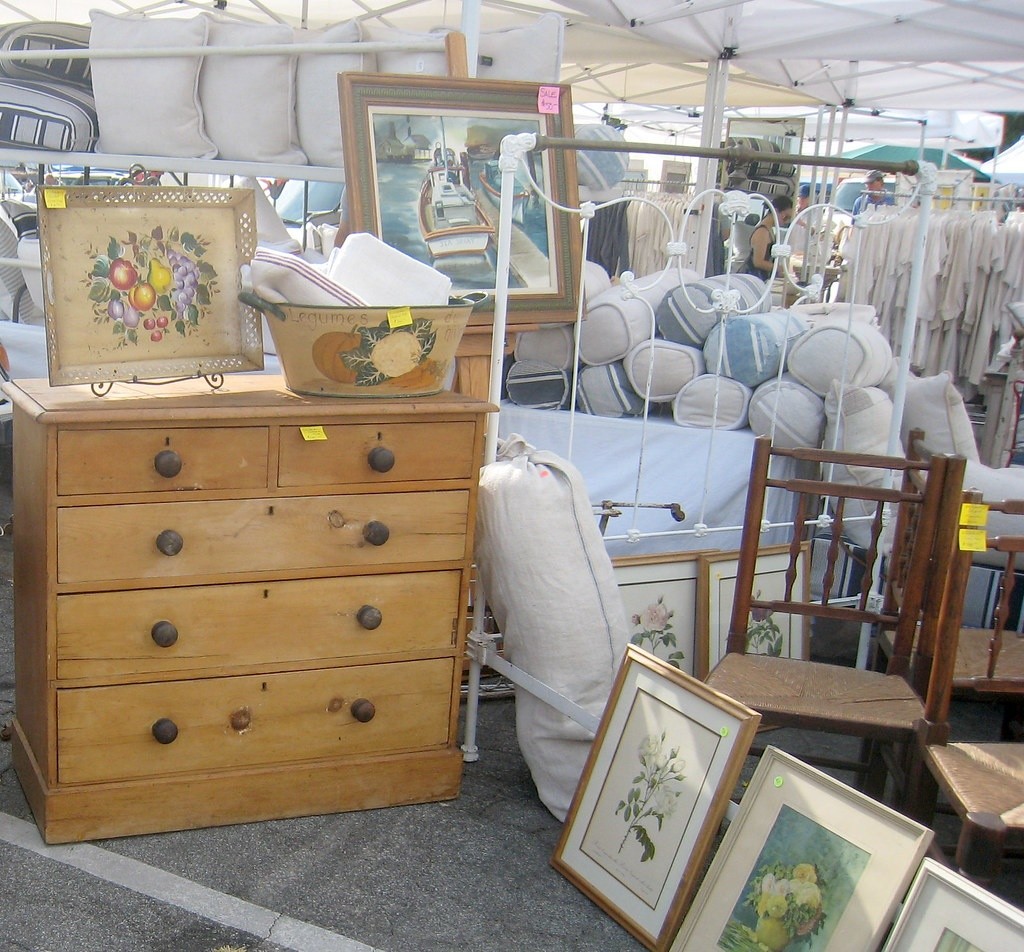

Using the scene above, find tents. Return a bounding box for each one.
[0,0,1024,284]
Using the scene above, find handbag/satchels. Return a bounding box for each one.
[734,255,768,283]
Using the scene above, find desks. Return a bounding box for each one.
[810,522,1024,657]
[500,399,825,557]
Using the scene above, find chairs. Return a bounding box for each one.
[701,426,1024,890]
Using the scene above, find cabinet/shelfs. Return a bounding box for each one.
[0,375,501,844]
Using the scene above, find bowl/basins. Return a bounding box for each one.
[238,290,486,398]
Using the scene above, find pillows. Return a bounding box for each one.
[505,260,1024,570]
[722,137,797,196]
[199,12,310,166]
[428,11,565,83]
[291,16,377,168]
[369,26,450,76]
[574,124,631,189]
[0,20,92,89]
[0,76,100,152]
[88,6,218,159]
[223,175,341,355]
[0,199,47,331]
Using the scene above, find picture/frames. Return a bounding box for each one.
[667,744,935,952]
[698,540,810,734]
[337,72,587,327]
[882,856,1024,952]
[34,184,265,387]
[549,643,762,952]
[609,548,720,679]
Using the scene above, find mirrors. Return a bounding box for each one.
[719,116,806,204]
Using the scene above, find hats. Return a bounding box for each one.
[797,185,811,197]
[861,168,887,185]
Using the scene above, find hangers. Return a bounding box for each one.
[868,187,1024,219]
[623,178,695,201]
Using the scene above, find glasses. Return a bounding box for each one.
[778,210,791,221]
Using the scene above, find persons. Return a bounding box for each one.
[734,195,793,284]
[852,170,895,225]
[795,185,812,213]
